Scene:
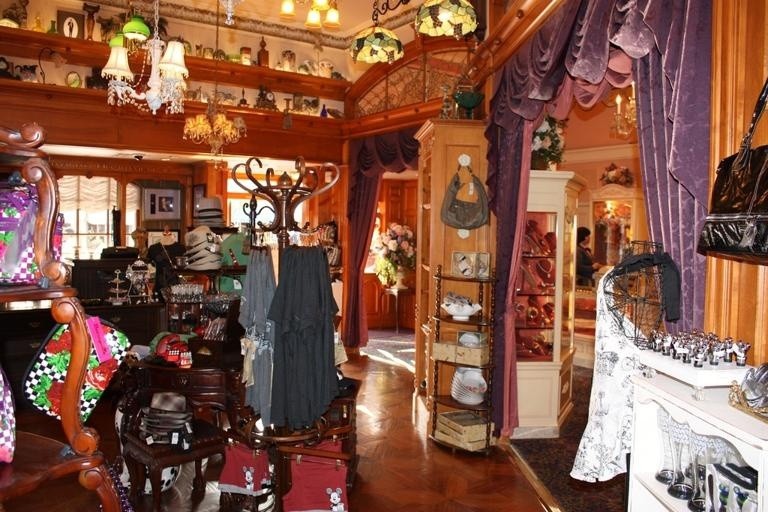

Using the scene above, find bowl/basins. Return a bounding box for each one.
[441,303,482,321]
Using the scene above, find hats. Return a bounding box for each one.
[137,392,194,447]
[192,198,226,227]
[183,225,225,271]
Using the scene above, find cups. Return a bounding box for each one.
[163,283,205,302]
[657,409,745,511]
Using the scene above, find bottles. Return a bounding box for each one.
[48,20,58,33]
[203,48,213,60]
[33,16,46,34]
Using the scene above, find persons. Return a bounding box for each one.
[575,226,600,288]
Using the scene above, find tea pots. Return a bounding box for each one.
[306,60,333,78]
[15,65,39,83]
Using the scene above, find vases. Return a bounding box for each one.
[387,270,409,291]
[528,150,551,169]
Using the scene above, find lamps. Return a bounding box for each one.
[193,195,225,217]
[413,1,480,39]
[611,79,641,141]
[346,0,409,66]
[0,1,351,135]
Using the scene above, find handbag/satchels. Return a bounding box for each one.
[1,178,65,286]
[697,143,767,265]
[20,315,130,426]
[440,164,490,229]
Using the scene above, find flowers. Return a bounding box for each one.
[528,111,568,158]
[376,220,416,283]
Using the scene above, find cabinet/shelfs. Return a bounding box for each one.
[509,167,585,439]
[84,303,165,348]
[3,316,54,401]
[626,372,767,508]
[410,116,488,441]
[423,267,506,460]
[0,119,131,512]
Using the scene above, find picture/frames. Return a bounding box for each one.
[141,186,182,223]
[144,228,181,247]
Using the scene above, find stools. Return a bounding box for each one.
[124,418,229,509]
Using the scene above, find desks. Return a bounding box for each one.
[115,359,287,512]
[376,288,416,336]
